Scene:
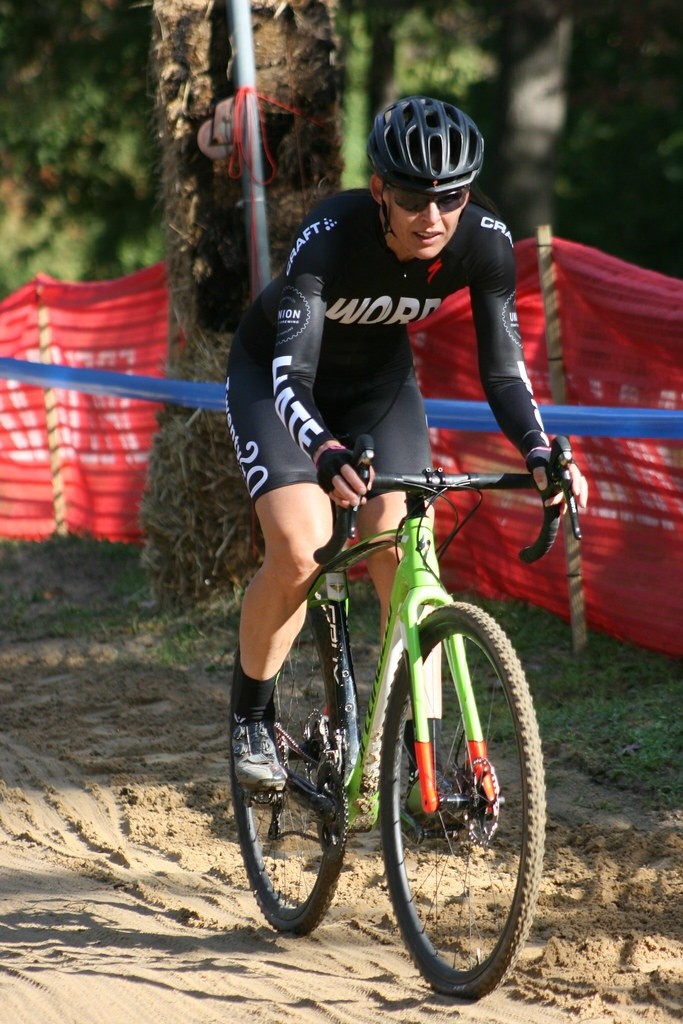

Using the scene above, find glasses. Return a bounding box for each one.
[381,183,472,213]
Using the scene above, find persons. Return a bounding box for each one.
[223,100,588,858]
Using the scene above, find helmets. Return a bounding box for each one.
[366,94,484,192]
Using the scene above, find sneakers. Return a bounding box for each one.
[409,769,454,810]
[229,711,288,791]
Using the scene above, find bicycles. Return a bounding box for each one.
[227,433,585,1007]
